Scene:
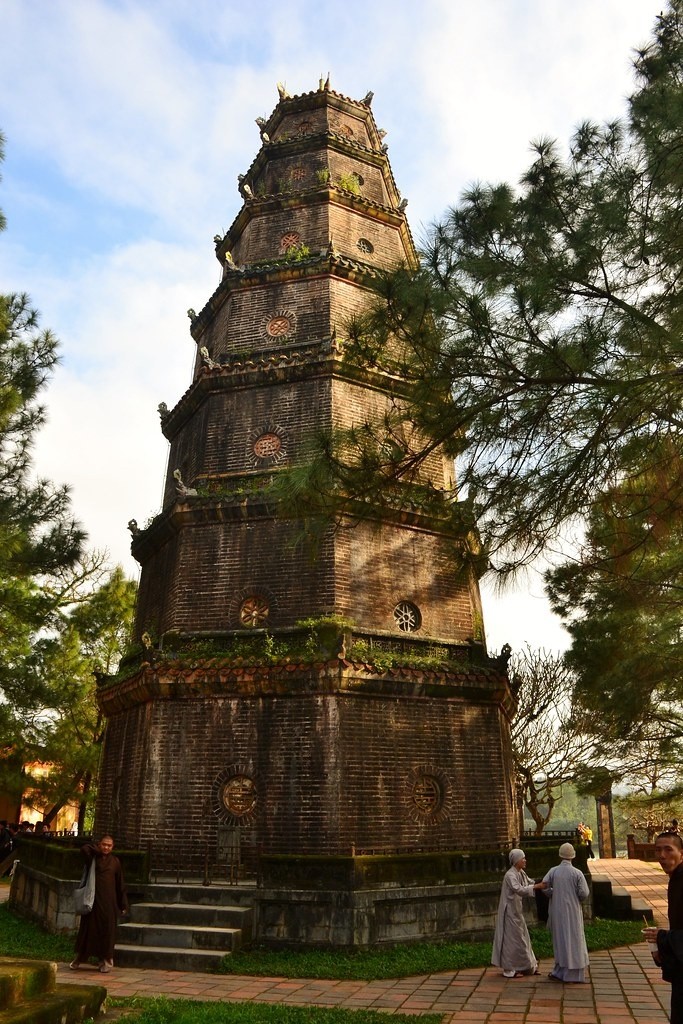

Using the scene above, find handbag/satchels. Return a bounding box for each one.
[72,858,96,915]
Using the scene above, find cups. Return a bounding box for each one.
[641,927,658,952]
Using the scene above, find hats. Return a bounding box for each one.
[559,843,575,859]
[509,849,525,865]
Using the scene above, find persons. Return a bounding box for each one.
[491,849,549,978]
[541,843,590,983]
[642,819,683,1024]
[578,821,596,861]
[0,820,51,878]
[69,835,128,973]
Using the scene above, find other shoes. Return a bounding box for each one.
[514,973,523,978]
[534,970,541,975]
[70,961,79,969]
